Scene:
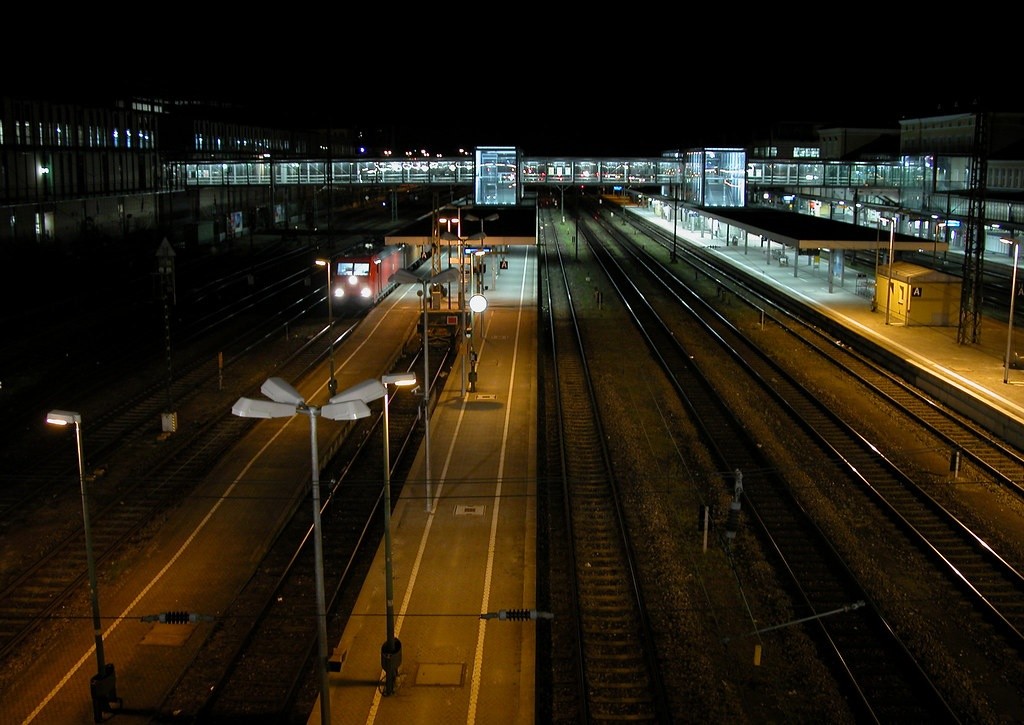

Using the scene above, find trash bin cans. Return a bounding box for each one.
[732,236,738,247]
[480,262,486,273]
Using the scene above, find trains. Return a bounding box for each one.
[328,203,465,314]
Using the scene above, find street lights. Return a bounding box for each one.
[314,258,339,398]
[464,214,499,330]
[230,374,388,725]
[438,217,459,311]
[878,217,895,325]
[389,266,461,515]
[440,230,486,398]
[380,370,418,697]
[45,406,119,725]
[999,235,1020,384]
